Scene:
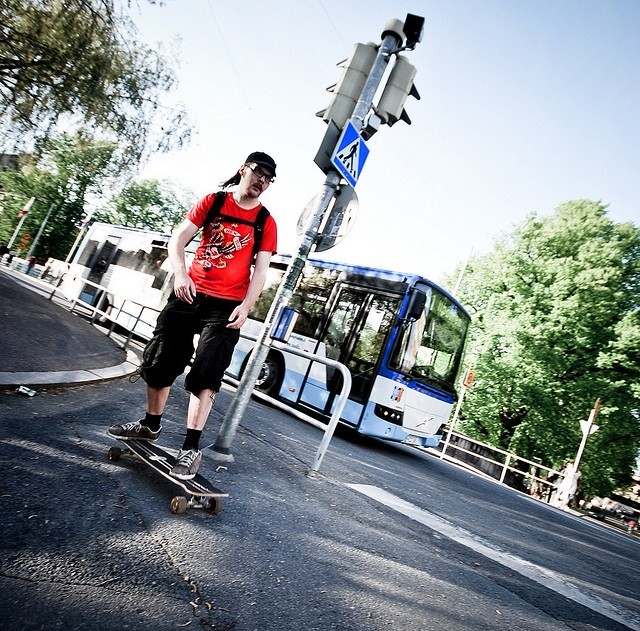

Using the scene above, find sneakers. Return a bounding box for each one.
[169,448,202,480]
[107,419,162,441]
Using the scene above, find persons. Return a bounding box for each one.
[106,150,279,481]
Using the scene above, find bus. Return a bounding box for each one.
[60,220,471,447]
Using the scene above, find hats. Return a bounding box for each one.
[245,151,276,178]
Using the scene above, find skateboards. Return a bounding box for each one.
[109,439,229,516]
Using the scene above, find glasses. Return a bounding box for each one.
[245,164,275,183]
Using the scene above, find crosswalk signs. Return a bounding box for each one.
[329,118,371,188]
